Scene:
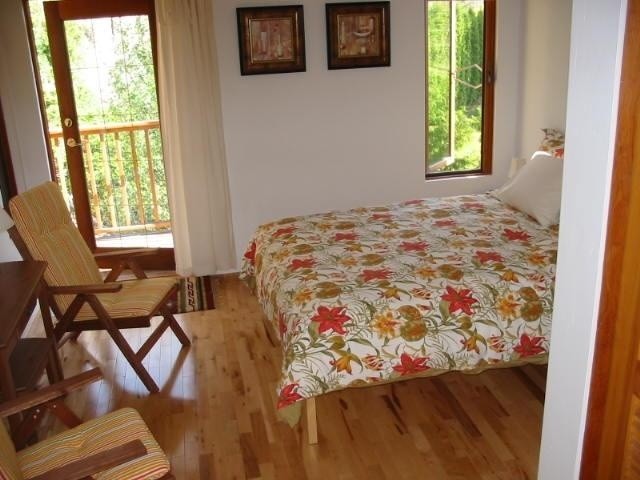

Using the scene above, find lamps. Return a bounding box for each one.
[506,155,528,180]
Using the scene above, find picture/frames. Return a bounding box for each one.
[235,4,307,77]
[325,0,391,71]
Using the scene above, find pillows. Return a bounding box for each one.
[536,125,568,161]
[490,148,566,229]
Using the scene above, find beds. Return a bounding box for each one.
[237,193,559,445]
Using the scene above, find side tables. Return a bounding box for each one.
[0,259,70,437]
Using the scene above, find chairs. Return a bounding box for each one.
[0,407,172,480]
[4,182,191,394]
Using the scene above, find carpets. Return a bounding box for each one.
[152,273,218,318]
